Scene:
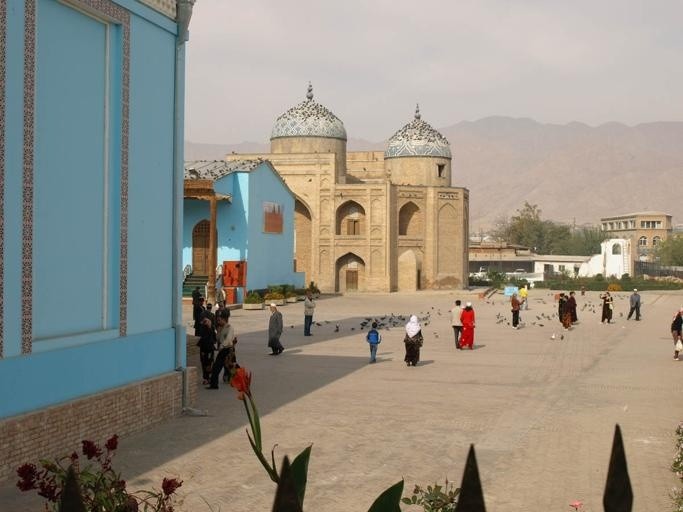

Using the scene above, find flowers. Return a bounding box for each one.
[567,499,584,512]
[228,362,312,510]
[12,431,189,511]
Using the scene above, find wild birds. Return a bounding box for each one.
[310,320,340,332]
[351,327,355,331]
[291,325,294,328]
[359,306,442,331]
[182,150,265,181]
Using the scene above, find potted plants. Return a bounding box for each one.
[241,280,320,310]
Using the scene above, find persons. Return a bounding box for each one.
[558,291,577,331]
[265,303,284,355]
[510,292,524,330]
[457,301,476,350]
[599,292,614,323]
[449,300,463,348]
[626,288,641,321]
[671,309,683,361]
[402,315,424,366]
[518,284,528,309]
[365,322,382,363]
[189,286,239,390]
[303,290,316,336]
